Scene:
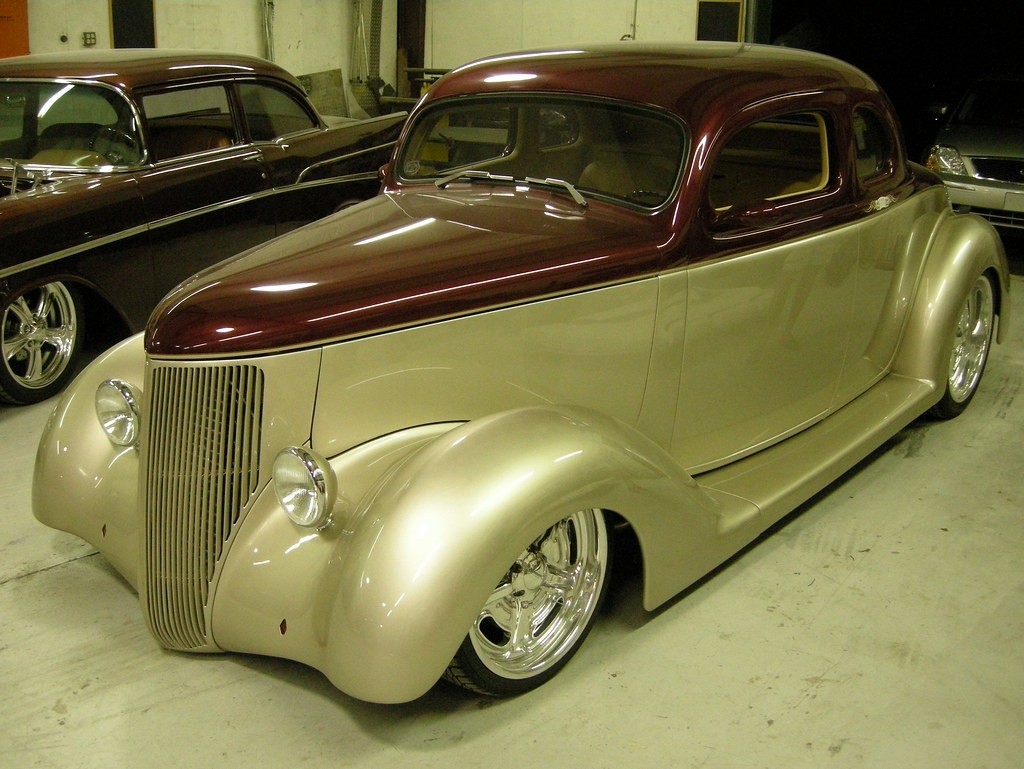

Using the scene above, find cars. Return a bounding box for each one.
[919,81,1024,239]
[0,48,407,407]
[30,41,1014,706]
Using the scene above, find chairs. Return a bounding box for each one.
[31,122,231,160]
[578,158,817,208]
